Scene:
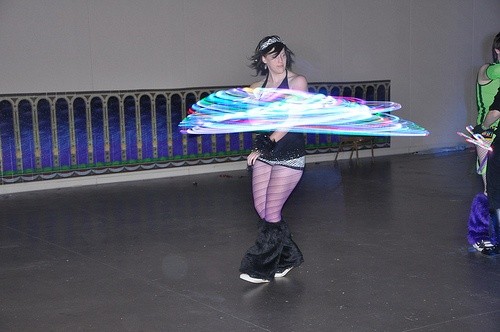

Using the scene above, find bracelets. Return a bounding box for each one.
[253,134,276,153]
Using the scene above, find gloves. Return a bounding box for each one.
[471,123,484,140]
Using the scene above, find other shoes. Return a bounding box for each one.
[473,239,496,254]
[274,259,302,278]
[240,272,271,284]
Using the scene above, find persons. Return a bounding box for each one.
[467,32,500,255]
[238,36,307,283]
